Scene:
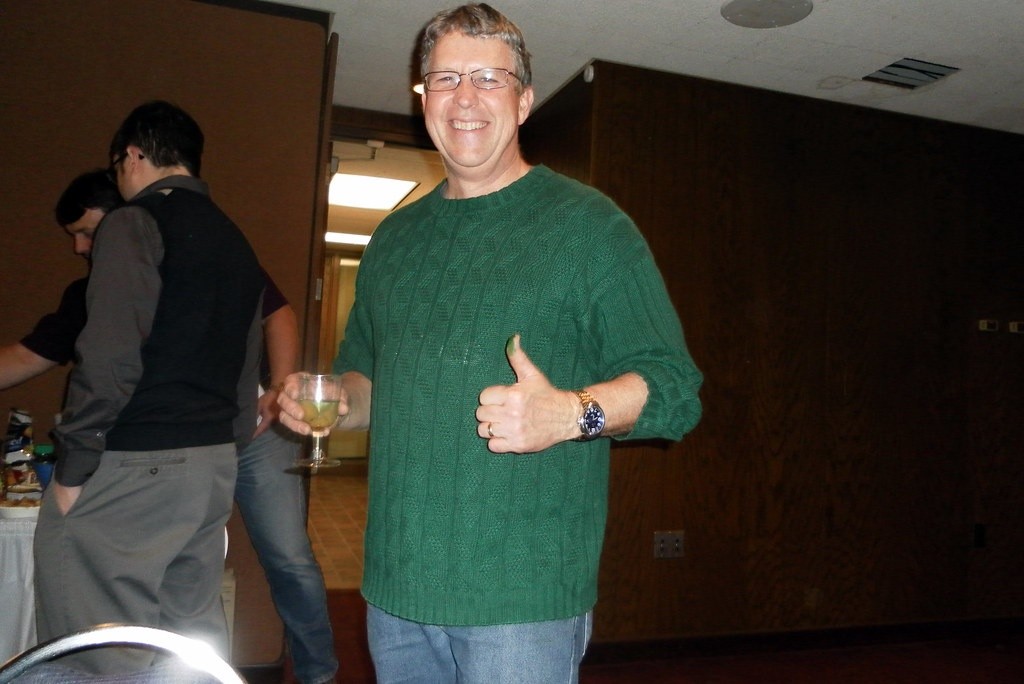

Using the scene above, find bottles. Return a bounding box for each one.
[34,445,55,492]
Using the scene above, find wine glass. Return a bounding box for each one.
[294,375,342,467]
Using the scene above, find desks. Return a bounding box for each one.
[0,517,38,665]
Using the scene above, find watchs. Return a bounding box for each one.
[571,387,605,441]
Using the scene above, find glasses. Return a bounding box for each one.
[416,68,525,92]
[105,153,145,180]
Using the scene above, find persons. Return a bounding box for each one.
[276,3,702,684]
[35,101,262,684]
[0,168,339,684]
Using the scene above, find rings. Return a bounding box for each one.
[488,424,494,437]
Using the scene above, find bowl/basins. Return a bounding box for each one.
[0,507,40,519]
